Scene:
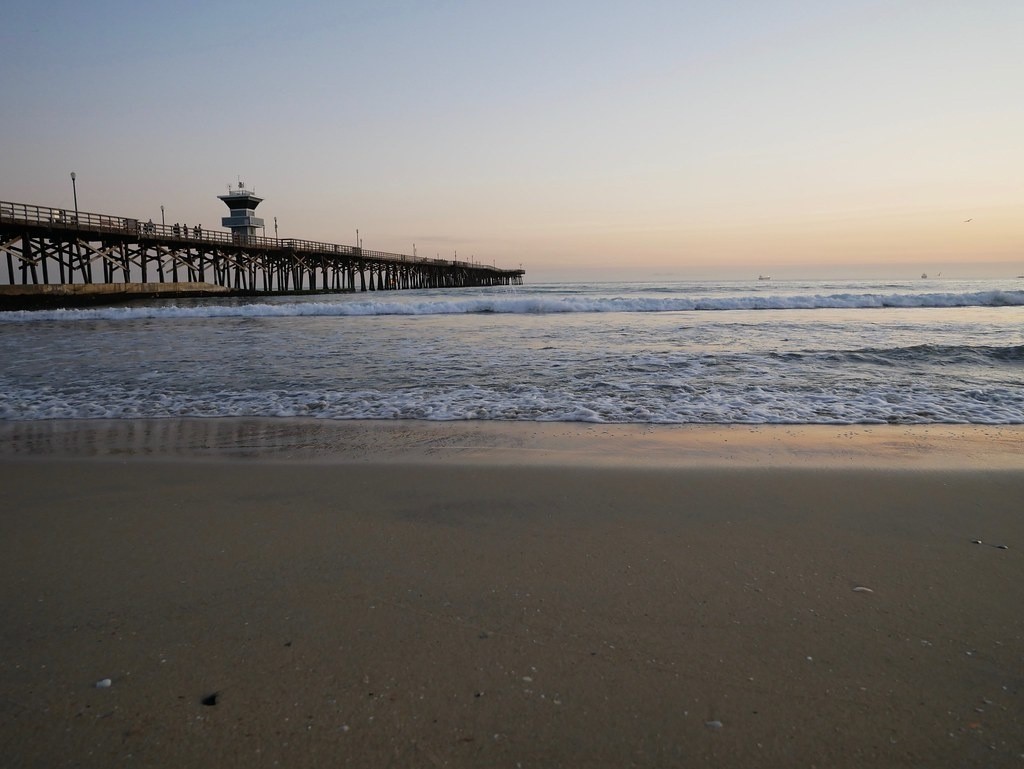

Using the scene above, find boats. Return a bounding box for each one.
[921,271,927,278]
[759,275,770,281]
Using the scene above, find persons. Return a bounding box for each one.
[147,218,155,235]
[173,222,202,239]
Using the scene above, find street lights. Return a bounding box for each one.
[436,251,523,269]
[274,217,279,247]
[412,243,417,255]
[356,229,363,248]
[161,206,165,234]
[70,172,79,225]
